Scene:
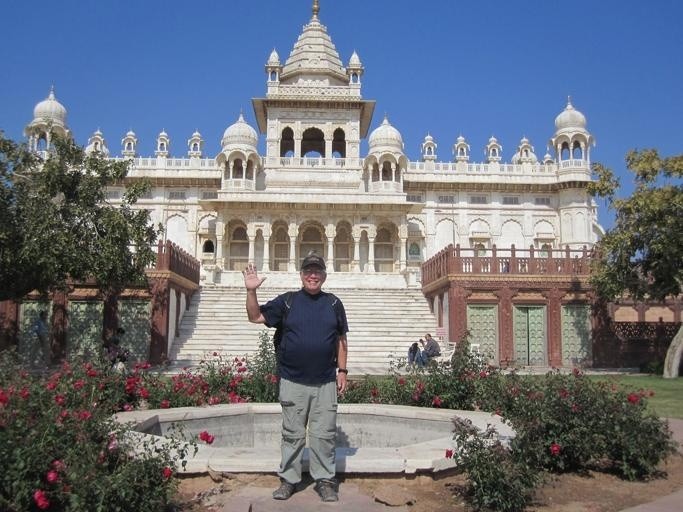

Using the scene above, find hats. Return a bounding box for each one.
[301,255,326,269]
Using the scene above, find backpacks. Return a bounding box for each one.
[273,291,341,351]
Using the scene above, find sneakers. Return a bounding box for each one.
[272,480,296,500]
[313,479,340,503]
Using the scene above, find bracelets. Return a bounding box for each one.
[337,368,348,375]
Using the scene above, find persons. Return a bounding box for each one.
[239,251,352,503]
[30,310,53,370]
[419,333,440,365]
[407,343,423,367]
[107,326,125,354]
